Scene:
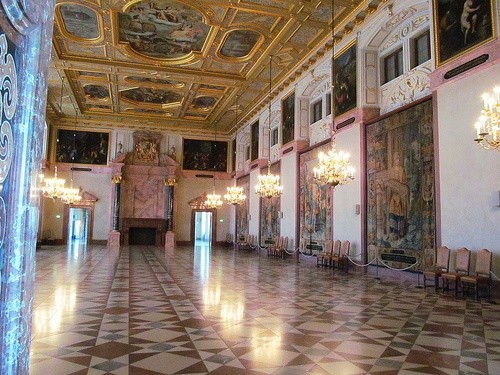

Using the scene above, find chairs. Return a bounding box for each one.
[267,236,288,260]
[237,234,254,254]
[220,233,233,248]
[317,239,350,273]
[423,246,493,302]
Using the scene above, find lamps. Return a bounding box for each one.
[254,54,281,200]
[473,86,500,154]
[313,0,355,190]
[41,77,82,205]
[203,120,224,210]
[223,94,247,206]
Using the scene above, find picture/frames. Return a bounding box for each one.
[281,87,296,149]
[180,136,231,174]
[432,0,497,69]
[332,36,358,122]
[53,126,111,167]
